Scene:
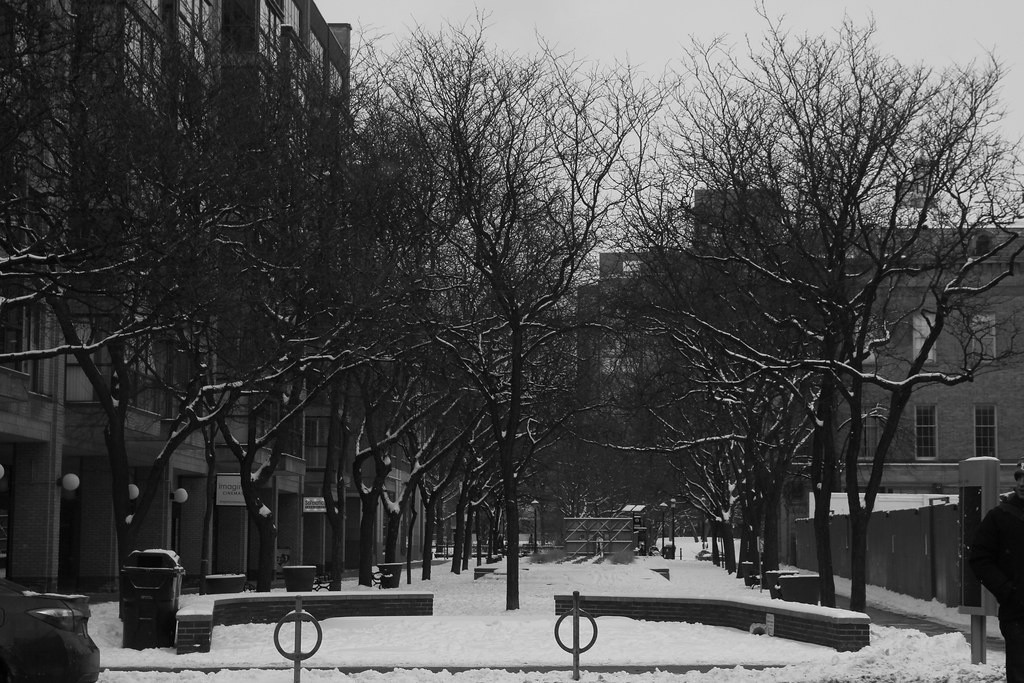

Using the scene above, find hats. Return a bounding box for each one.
[1014,455,1024,480]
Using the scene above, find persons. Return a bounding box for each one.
[972,456,1024,683]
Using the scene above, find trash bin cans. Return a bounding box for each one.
[120,548,186,651]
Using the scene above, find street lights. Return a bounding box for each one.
[670,499,676,556]
[660,503,669,555]
[530,500,541,552]
